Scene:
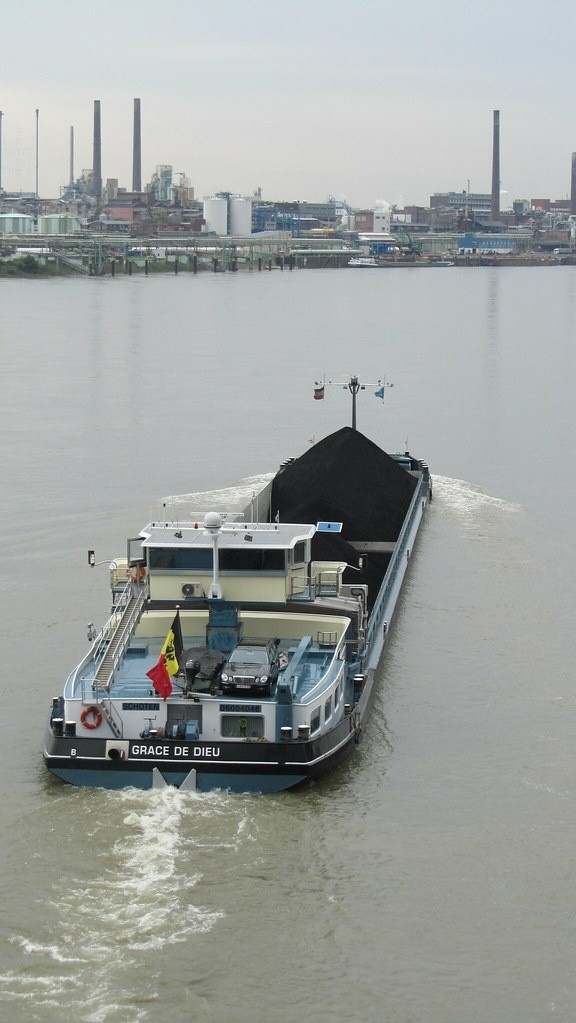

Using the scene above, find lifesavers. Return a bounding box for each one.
[126,566,146,581]
[79,706,103,730]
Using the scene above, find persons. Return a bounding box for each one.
[238,717,247,737]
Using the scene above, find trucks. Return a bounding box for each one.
[554,248,572,253]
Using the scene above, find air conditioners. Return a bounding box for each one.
[181,582,203,597]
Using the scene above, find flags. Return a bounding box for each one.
[157,613,184,677]
[146,654,173,700]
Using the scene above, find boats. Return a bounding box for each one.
[44,371,433,794]
[349,256,379,267]
